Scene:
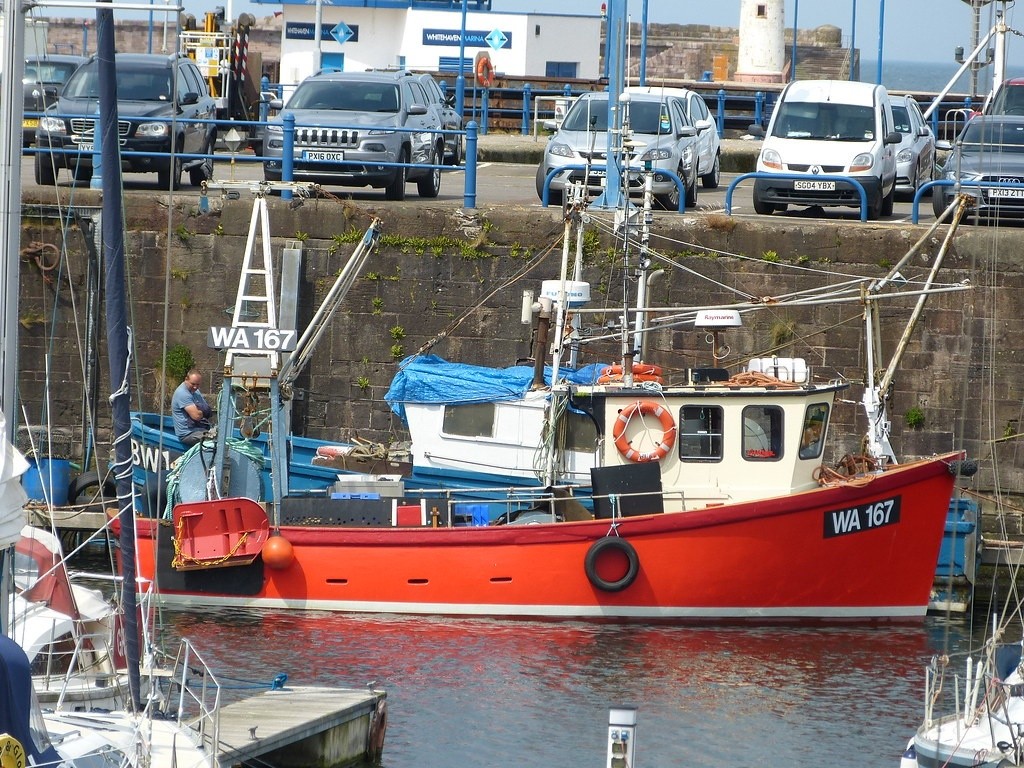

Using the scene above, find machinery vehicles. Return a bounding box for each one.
[169,5,270,160]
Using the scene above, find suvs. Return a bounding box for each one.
[967,78,1024,122]
[33,53,217,192]
[262,66,445,200]
[873,95,936,203]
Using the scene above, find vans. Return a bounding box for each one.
[751,80,905,221]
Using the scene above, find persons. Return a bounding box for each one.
[170,368,213,445]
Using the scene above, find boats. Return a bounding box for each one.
[87,161,972,626]
[1,1,1024,768]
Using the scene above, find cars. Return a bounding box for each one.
[620,87,723,189]
[536,90,701,214]
[934,116,1024,225]
[375,74,464,163]
[22,53,89,150]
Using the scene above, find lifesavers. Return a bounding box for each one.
[600,360,664,385]
[585,534,638,591]
[615,402,679,465]
